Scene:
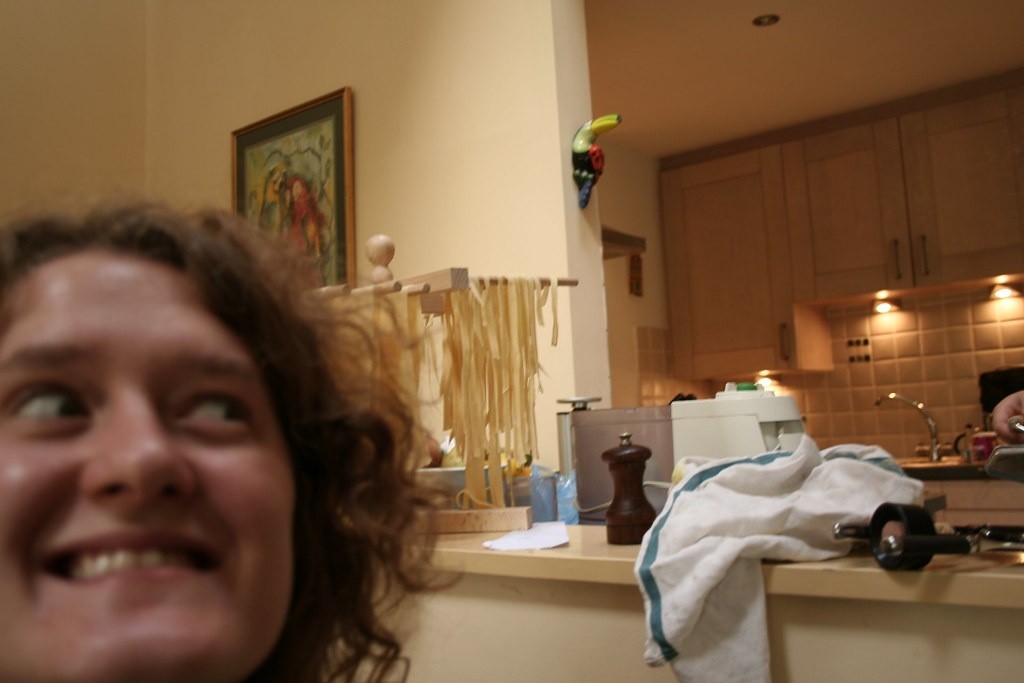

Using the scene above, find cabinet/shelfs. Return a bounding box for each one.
[792,90,1024,310]
[657,127,835,380]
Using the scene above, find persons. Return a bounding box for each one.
[992,390,1024,444]
[2,197,439,683]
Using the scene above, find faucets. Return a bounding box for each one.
[874,394,942,462]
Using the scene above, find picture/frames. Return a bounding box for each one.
[228,85,357,290]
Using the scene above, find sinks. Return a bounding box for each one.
[897,459,949,465]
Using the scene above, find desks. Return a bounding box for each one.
[316,504,1024,683]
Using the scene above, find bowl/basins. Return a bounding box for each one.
[416,464,508,492]
